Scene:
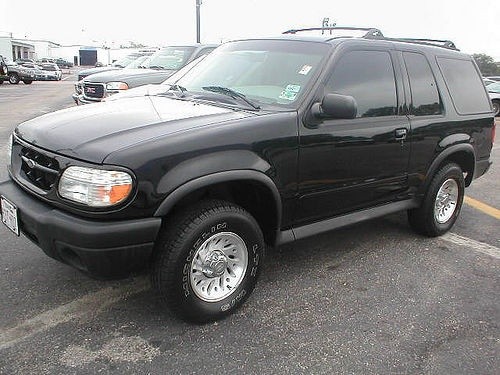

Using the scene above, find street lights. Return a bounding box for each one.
[196,0,203,44]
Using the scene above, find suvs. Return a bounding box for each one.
[0,26,495,326]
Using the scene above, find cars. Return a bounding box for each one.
[72,43,219,106]
[77,52,155,82]
[124,56,151,69]
[483,79,500,117]
[0,55,37,84]
[95,62,104,67]
[14,57,73,81]
